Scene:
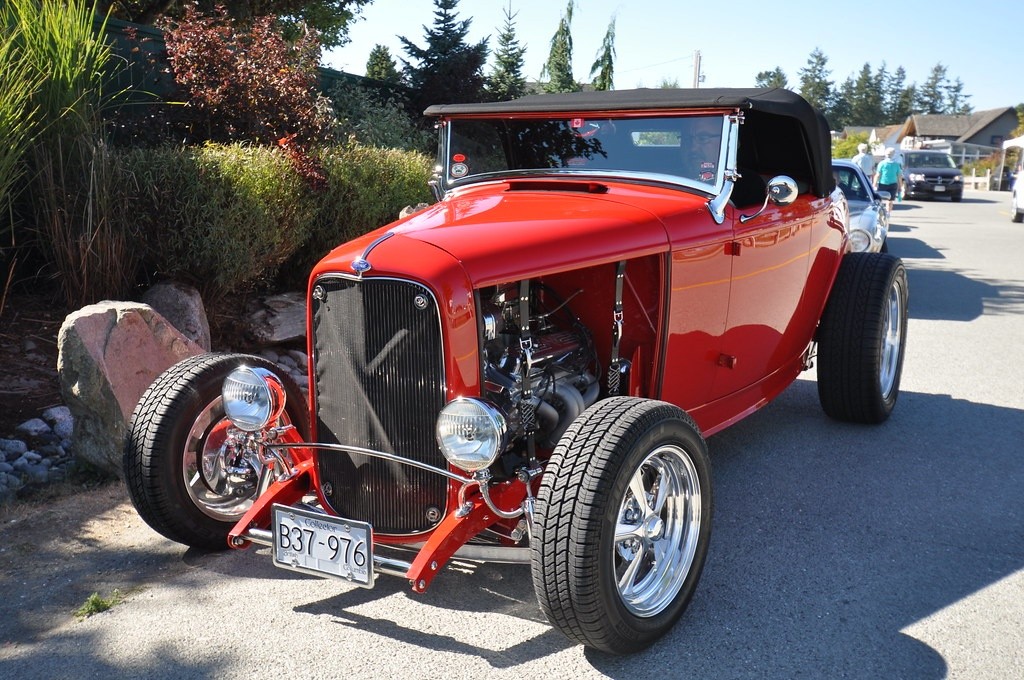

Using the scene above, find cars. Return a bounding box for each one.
[1012,170,1024,223]
[897,149,963,201]
[829,159,893,253]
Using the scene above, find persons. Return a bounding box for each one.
[1010,164,1022,198]
[667,116,767,207]
[852,141,875,191]
[875,145,905,212]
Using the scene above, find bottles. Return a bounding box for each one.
[898,191,902,203]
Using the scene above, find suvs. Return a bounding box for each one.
[121,89,908,657]
